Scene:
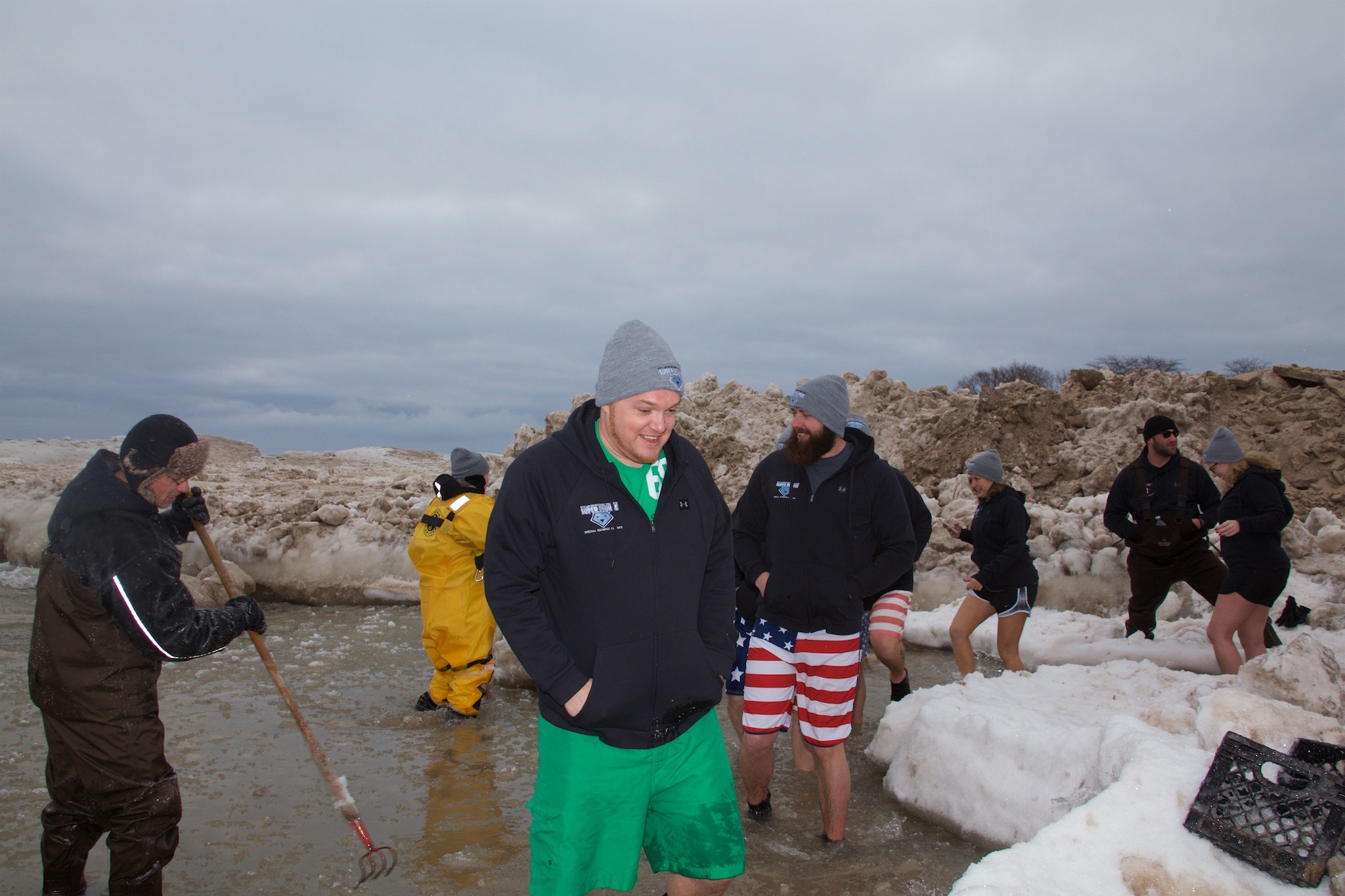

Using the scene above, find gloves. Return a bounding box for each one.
[1275,595,1311,627]
[224,594,268,635]
[173,487,211,535]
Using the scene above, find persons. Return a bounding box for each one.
[842,413,935,722]
[1202,425,1294,675]
[725,425,816,775]
[408,448,495,719]
[940,449,1040,684]
[731,374,917,845]
[484,319,744,896]
[1103,416,1285,648]
[28,414,268,896]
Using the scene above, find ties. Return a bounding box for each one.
[845,415,871,436]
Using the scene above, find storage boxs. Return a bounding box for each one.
[1183,730,1345,889]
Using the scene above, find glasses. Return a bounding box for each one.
[1209,463,1219,473]
[1158,429,1180,438]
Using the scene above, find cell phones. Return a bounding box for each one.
[942,521,958,534]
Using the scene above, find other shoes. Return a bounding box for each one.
[890,666,910,701]
[746,789,772,820]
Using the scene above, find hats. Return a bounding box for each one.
[120,413,210,505]
[596,319,684,407]
[967,448,1003,483]
[1143,415,1178,443]
[451,448,489,487]
[1203,425,1244,463]
[777,425,793,450]
[789,374,850,439]
